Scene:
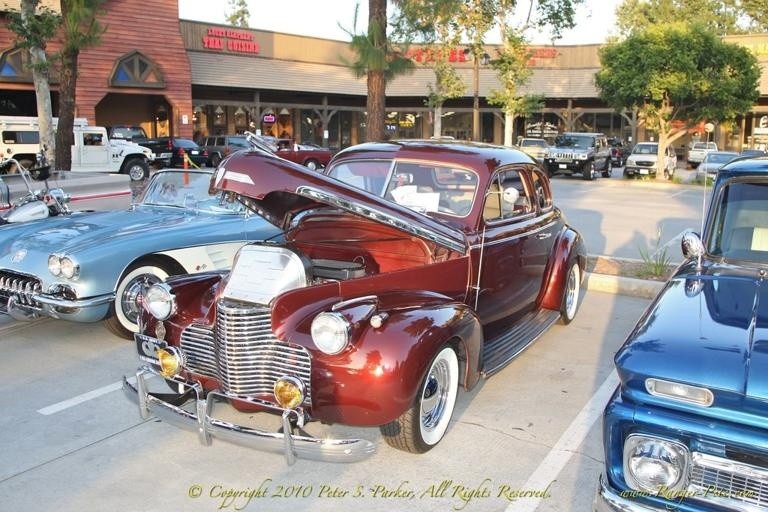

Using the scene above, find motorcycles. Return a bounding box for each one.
[1,155,96,225]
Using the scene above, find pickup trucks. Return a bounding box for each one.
[103,123,334,175]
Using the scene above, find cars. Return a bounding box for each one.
[122,133,588,458]
[1,168,287,340]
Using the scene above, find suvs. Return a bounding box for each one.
[598,155,768,509]
[510,132,768,192]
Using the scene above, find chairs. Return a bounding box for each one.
[727,206,767,251]
[461,186,508,220]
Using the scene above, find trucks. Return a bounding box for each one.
[1,113,157,184]
